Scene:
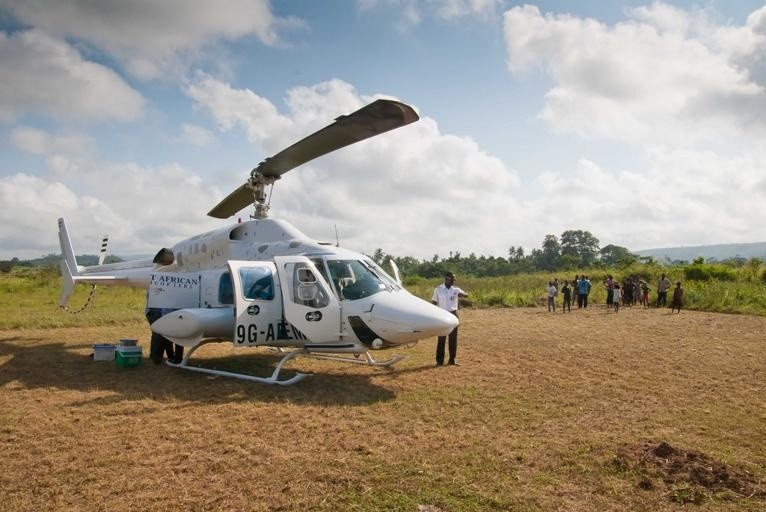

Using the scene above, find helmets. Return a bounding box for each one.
[445,271,457,281]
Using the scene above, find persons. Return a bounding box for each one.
[546,273,652,313]
[144,245,187,366]
[432,271,470,366]
[656,273,673,307]
[671,281,684,314]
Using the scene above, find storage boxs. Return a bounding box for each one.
[92,344,118,362]
[117,345,142,371]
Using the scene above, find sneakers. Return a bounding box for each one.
[437,362,461,367]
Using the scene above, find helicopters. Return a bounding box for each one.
[56,99,459,385]
